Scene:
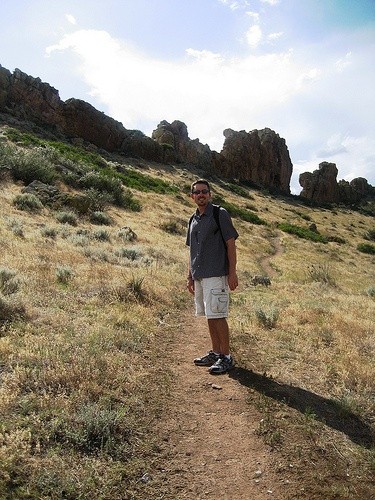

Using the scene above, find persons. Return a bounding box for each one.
[186,179,239,374]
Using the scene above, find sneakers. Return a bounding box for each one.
[209,353,233,373]
[194,350,221,366]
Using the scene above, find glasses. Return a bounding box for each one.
[192,189,209,194]
